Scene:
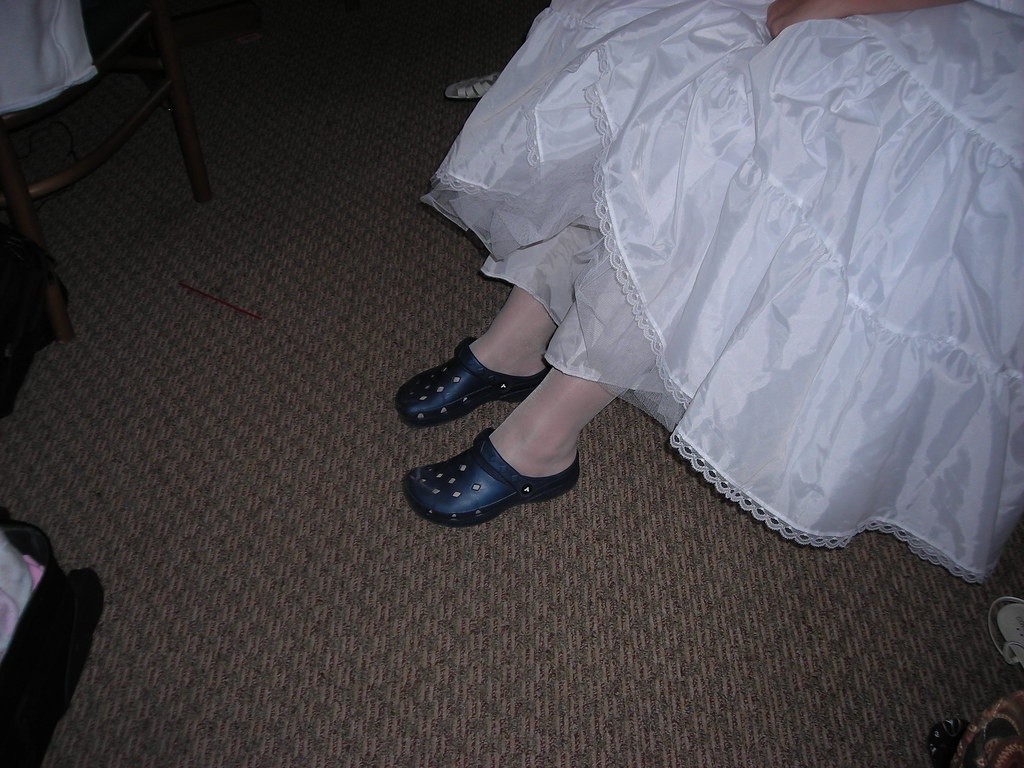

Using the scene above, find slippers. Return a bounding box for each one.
[402,429,580,528]
[396,336,549,429]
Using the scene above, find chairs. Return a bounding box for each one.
[0,0,213,346]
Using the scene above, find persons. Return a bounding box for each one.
[394,0,1024,583]
